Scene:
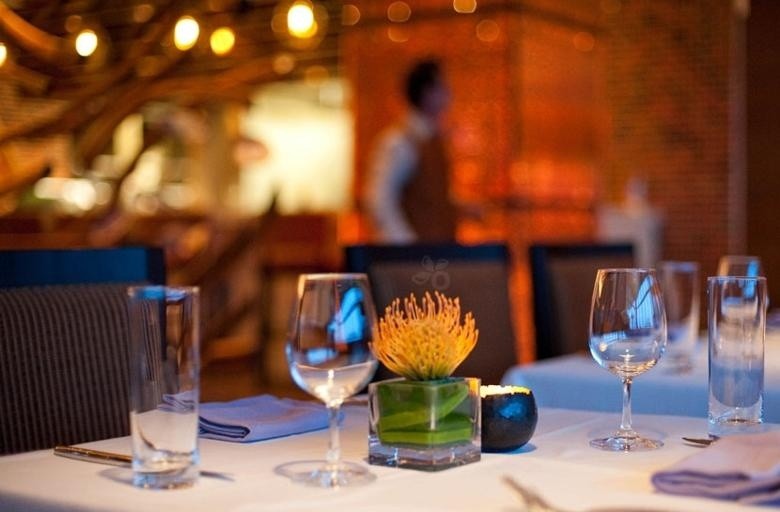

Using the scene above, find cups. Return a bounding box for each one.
[654,262,703,373]
[705,252,768,435]
[125,282,202,491]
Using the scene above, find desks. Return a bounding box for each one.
[2,394,778,510]
[500,326,779,424]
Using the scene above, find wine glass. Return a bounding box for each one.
[284,272,382,489]
[584,267,668,451]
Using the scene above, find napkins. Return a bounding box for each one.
[193,397,343,443]
[650,431,778,505]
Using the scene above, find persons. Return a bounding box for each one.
[359,58,481,246]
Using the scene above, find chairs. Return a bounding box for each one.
[0,246,636,453]
